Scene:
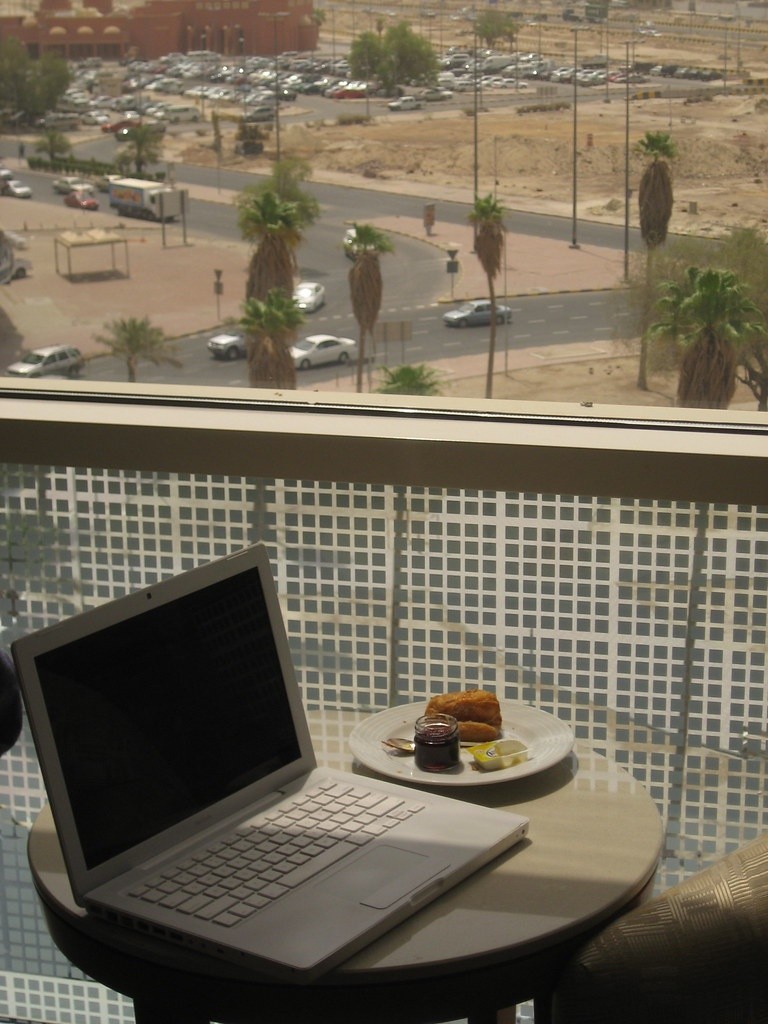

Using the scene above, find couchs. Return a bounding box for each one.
[550,833,768,1024]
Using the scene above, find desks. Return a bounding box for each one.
[28,708,667,1023]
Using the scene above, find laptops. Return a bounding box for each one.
[11,539,531,987]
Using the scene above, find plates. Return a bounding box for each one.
[348,698,575,786]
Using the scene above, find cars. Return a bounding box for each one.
[51,176,93,195]
[287,333,358,371]
[95,175,122,191]
[65,189,99,210]
[342,229,378,261]
[291,281,326,315]
[0,169,32,200]
[36,49,723,142]
[205,323,248,361]
[442,300,514,329]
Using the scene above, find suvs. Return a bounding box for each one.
[4,344,86,380]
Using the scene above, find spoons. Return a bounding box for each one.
[381,737,488,751]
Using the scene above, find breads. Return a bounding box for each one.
[424,689,502,739]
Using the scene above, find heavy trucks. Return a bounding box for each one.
[108,178,190,224]
